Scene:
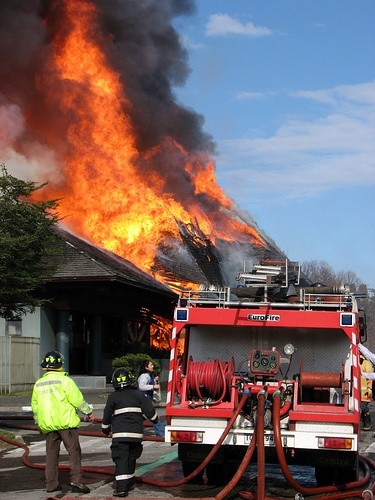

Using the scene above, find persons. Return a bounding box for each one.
[31,351,96,494]
[101,368,160,498]
[350,350,374,432]
[136,357,167,419]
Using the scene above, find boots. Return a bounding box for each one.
[360,410,373,431]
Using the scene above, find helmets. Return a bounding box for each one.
[40,351,64,371]
[110,368,134,390]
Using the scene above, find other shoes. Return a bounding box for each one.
[47,485,63,492]
[113,491,128,497]
[71,482,90,493]
[130,485,135,490]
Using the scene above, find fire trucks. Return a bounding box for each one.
[164,259,368,486]
